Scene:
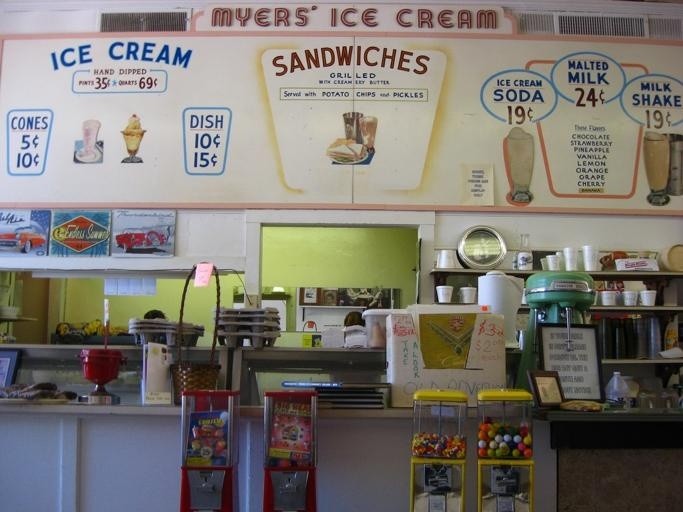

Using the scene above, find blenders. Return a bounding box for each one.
[512,270,596,407]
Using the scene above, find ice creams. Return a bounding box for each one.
[121,114,146,156]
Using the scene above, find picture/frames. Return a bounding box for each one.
[297,285,323,306]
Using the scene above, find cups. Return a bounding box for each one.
[435,249,457,268]
[343,112,378,149]
[503,134,536,205]
[593,290,657,306]
[82,120,101,155]
[436,286,453,303]
[460,286,477,303]
[636,387,680,410]
[642,133,683,206]
[539,245,599,272]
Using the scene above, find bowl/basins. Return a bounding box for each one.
[0,306,20,319]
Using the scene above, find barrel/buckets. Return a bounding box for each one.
[605,372,630,412]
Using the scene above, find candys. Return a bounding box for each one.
[478,416,533,458]
[412,432,466,458]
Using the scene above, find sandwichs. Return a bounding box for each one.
[329,143,365,161]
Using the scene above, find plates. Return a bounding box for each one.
[330,151,369,164]
[0,397,70,406]
[75,149,102,163]
[661,243,683,272]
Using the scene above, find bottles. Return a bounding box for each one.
[517,233,534,272]
[595,312,661,360]
[604,372,639,410]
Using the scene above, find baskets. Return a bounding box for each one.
[171,262,222,406]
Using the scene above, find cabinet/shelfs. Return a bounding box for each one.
[428,268,682,369]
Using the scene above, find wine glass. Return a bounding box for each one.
[77,348,129,404]
[119,130,146,164]
